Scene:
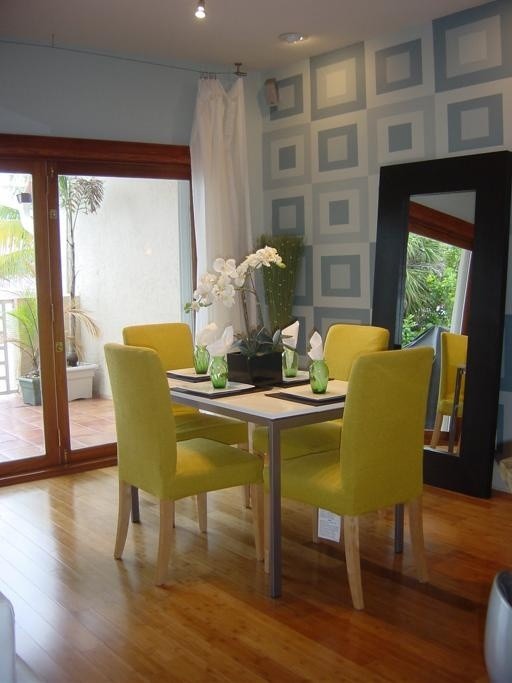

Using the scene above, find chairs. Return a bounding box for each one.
[264,345,434,611]
[254,323,391,543]
[483,569,512,683]
[121,323,251,532]
[103,342,265,587]
[429,331,468,448]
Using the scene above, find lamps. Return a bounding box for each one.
[195,0,206,20]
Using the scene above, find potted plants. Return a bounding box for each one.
[58,175,105,403]
[0,206,100,406]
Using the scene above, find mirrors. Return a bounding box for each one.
[372,149,511,499]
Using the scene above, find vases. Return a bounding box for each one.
[226,352,283,387]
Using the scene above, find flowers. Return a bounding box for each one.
[183,244,287,356]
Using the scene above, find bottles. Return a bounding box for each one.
[192,344,210,374]
[281,349,299,378]
[310,359,330,393]
[208,355,229,389]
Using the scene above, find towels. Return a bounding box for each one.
[307,330,328,391]
[195,325,219,370]
[280,320,300,373]
[206,325,234,376]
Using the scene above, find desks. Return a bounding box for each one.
[499,457,510,487]
